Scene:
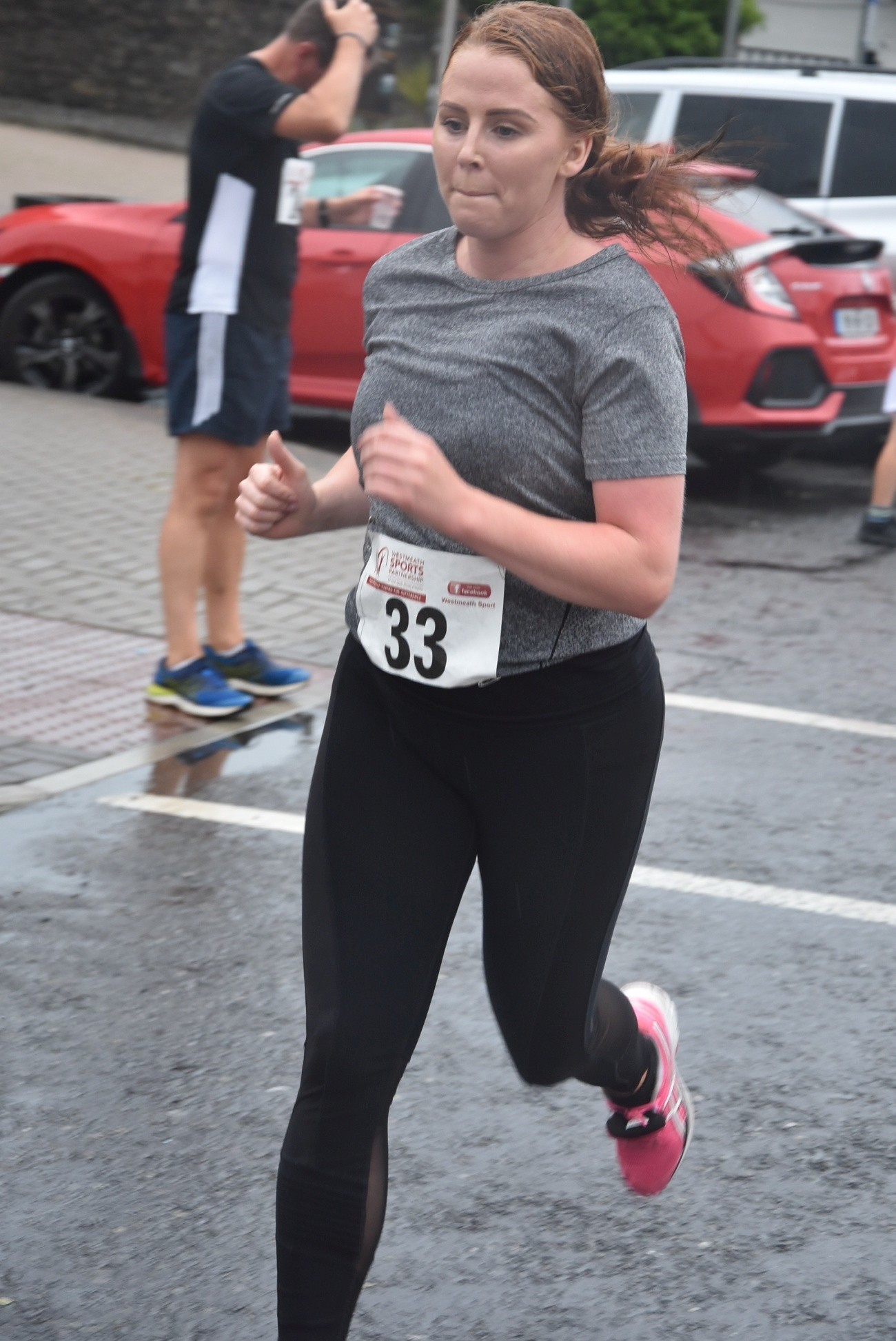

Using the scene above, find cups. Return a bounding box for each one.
[369,185,405,229]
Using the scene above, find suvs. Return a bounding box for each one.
[597,55,895,287]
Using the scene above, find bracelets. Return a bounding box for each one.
[337,32,374,60]
[318,198,330,230]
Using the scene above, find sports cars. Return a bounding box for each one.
[0,123,896,497]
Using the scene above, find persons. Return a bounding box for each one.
[855,368,896,547]
[233,2,693,1340]
[144,1,380,717]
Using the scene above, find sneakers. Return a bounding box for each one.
[146,656,253,718]
[200,636,311,696]
[605,980,693,1196]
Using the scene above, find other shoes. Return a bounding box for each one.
[858,508,896,546]
